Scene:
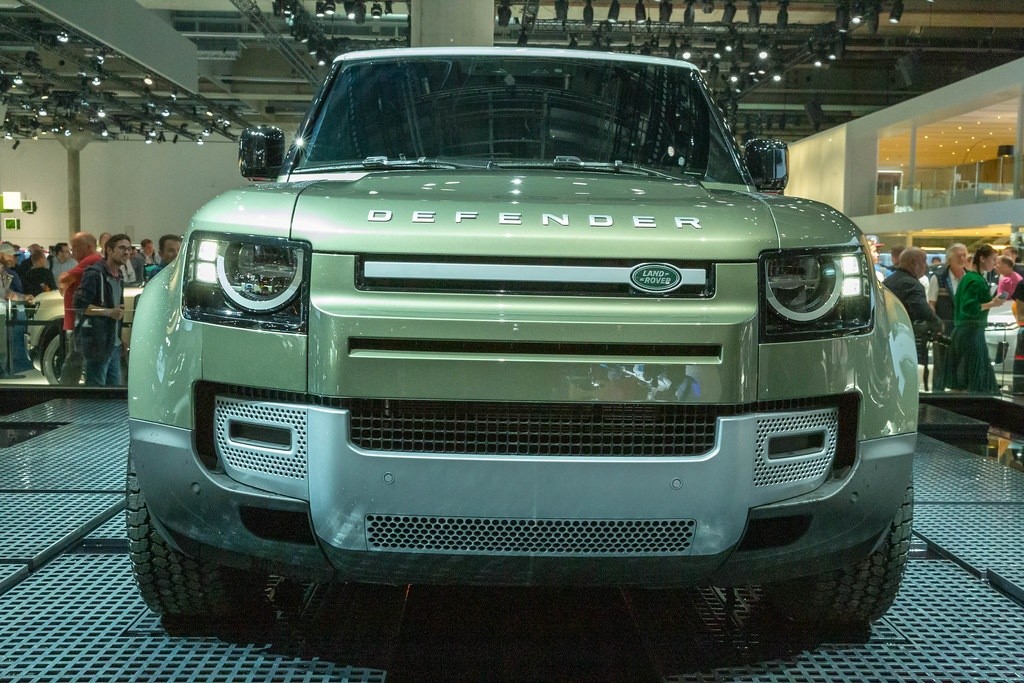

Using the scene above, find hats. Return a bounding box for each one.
[867,240,885,247]
[0,244,21,256]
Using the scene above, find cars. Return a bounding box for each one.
[25,261,163,384]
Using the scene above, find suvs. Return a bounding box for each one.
[121,45,920,634]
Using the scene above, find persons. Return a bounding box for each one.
[867,235,1024,397]
[0,232,183,385]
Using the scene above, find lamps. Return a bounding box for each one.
[721,0,737,25]
[668,32,846,93]
[863,11,879,35]
[834,0,850,32]
[581,0,594,26]
[684,2,695,28]
[554,0,570,21]
[0,0,393,144]
[888,0,904,25]
[659,0,673,25]
[607,0,621,23]
[746,0,762,27]
[635,0,646,24]
[955,138,1014,189]
[775,0,790,30]
[497,8,512,28]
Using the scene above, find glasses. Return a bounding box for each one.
[119,245,132,251]
